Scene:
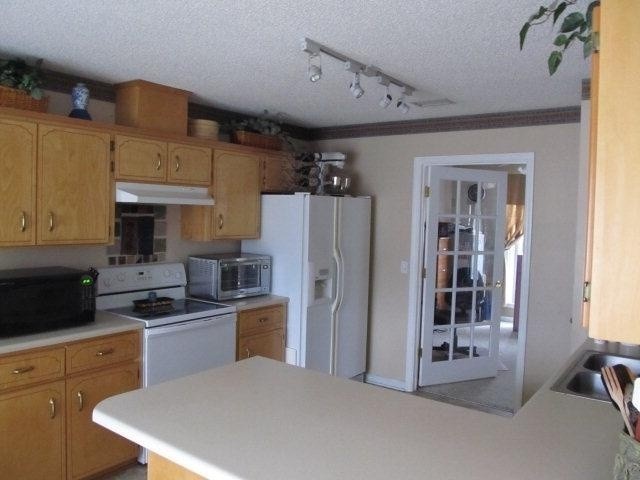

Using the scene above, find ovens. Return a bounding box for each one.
[136,313,237,464]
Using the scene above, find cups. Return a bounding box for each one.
[146,290,158,304]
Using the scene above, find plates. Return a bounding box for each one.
[130,296,173,311]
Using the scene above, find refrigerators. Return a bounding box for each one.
[241,192,372,385]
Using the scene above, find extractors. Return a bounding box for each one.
[115,182,217,206]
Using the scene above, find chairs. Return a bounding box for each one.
[434,265,487,359]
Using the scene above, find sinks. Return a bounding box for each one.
[550,369,612,405]
[577,350,640,378]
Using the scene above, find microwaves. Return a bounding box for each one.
[188,252,271,301]
[0,265,99,339]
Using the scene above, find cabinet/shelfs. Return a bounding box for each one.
[1,324,147,480]
[236,298,286,364]
[263,151,298,194]
[114,136,213,185]
[181,152,261,241]
[1,108,111,246]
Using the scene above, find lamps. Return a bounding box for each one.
[346,59,366,99]
[304,41,323,83]
[394,83,412,116]
[379,73,390,109]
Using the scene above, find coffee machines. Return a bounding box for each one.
[316,151,351,195]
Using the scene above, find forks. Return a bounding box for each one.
[601,364,637,438]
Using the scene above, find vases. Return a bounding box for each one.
[67,79,95,120]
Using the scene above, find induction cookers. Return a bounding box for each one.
[94,267,236,327]
